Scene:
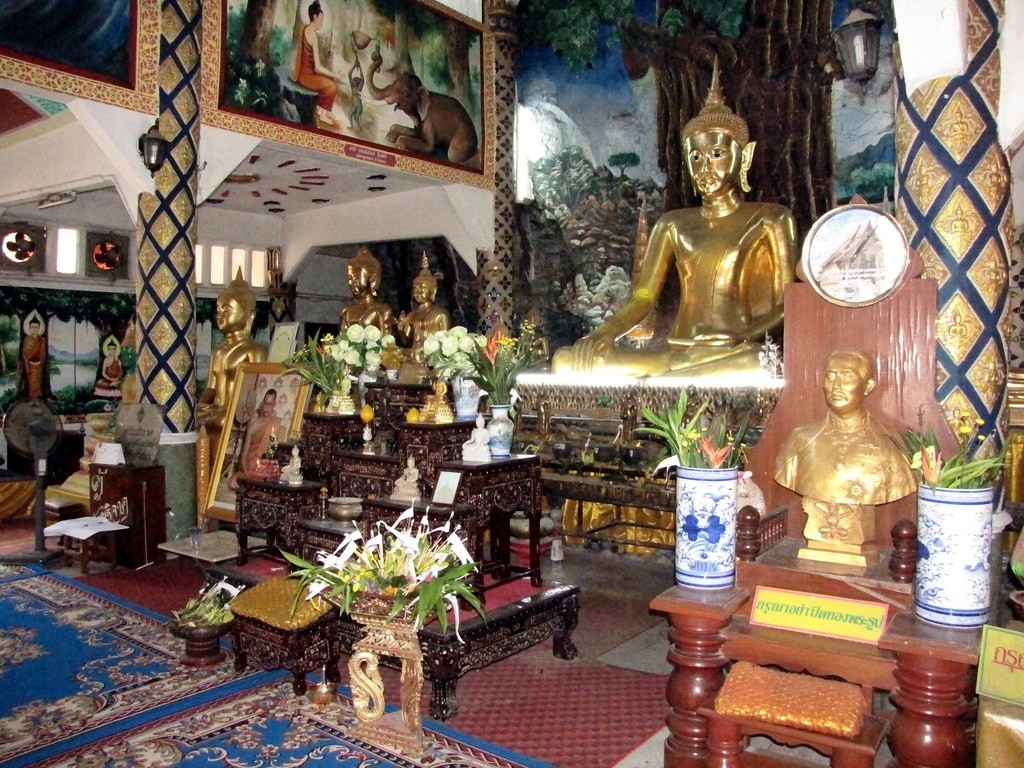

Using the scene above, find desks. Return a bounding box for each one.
[648,561,987,768]
[204,381,580,721]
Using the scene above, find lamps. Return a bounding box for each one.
[361,403,375,443]
[832,6,886,94]
[139,119,169,177]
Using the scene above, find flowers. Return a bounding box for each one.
[467,318,550,404]
[426,324,486,377]
[330,323,396,367]
[632,388,751,481]
[282,327,356,394]
[884,407,1016,495]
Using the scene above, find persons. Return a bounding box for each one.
[684,384,700,428]
[551,54,798,380]
[338,245,392,335]
[774,349,918,505]
[397,250,451,365]
[230,389,285,490]
[462,414,490,451]
[532,387,552,437]
[423,371,447,405]
[197,265,266,432]
[281,444,301,475]
[620,386,637,442]
[394,455,418,487]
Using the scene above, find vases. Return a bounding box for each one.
[357,366,382,411]
[326,397,357,414]
[452,377,487,417]
[674,465,739,590]
[914,484,995,629]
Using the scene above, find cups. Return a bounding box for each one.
[189,526,203,551]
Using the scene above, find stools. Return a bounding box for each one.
[695,685,886,768]
[41,497,119,574]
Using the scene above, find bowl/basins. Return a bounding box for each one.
[327,497,363,527]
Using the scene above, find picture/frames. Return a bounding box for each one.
[201,361,312,521]
[488,404,513,458]
